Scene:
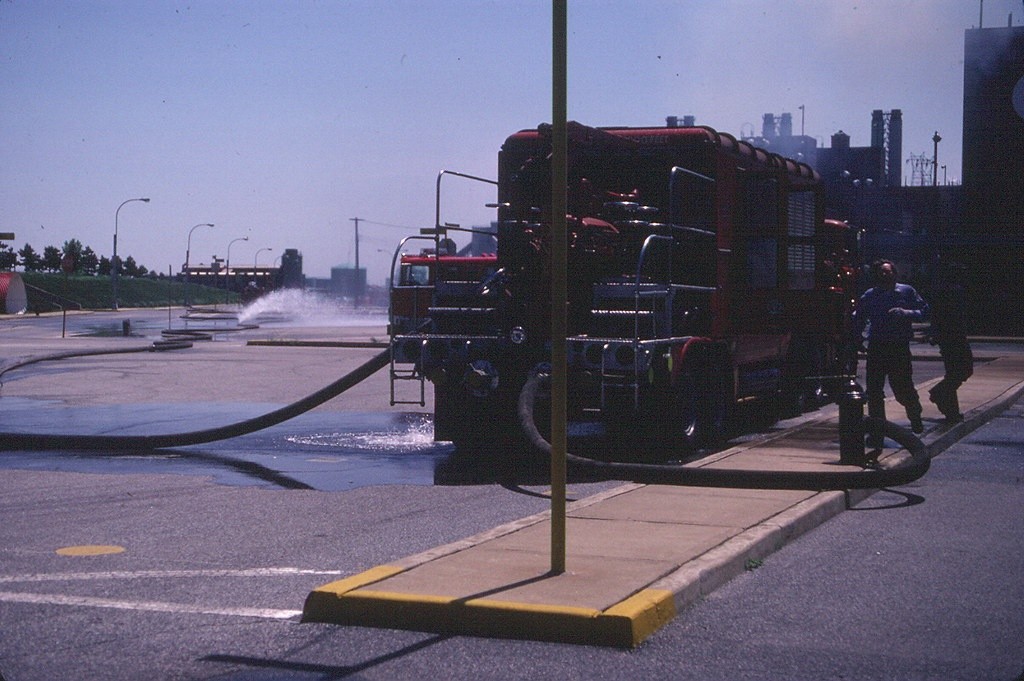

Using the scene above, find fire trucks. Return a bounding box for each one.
[387,121,867,457]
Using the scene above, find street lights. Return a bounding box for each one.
[184,224,214,306]
[254,248,272,282]
[932,131,942,186]
[798,104,804,136]
[112,198,150,309]
[226,238,249,304]
[941,165,946,185]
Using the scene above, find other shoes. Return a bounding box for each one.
[911,416,924,434]
[866,436,884,448]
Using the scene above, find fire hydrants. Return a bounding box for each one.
[835,378,869,465]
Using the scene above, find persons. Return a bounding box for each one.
[911,297,973,426]
[854,260,930,445]
